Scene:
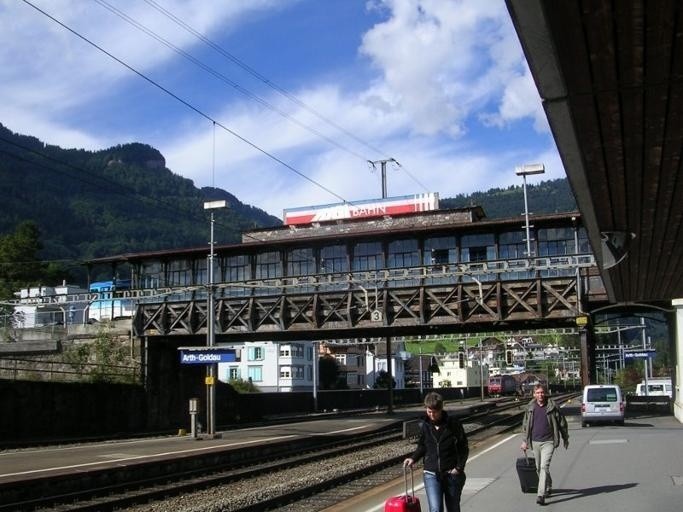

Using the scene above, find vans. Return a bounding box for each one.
[636,380,673,400]
[581,384,624,426]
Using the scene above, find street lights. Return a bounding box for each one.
[515,164,546,255]
[204,201,227,434]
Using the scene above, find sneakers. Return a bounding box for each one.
[536,491,551,504]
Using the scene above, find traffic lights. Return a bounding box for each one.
[458,352,465,369]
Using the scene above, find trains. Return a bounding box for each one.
[488,374,516,396]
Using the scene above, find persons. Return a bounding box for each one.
[520,383,569,505]
[403,392,469,512]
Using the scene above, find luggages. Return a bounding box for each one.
[384,463,421,512]
[516,449,540,494]
